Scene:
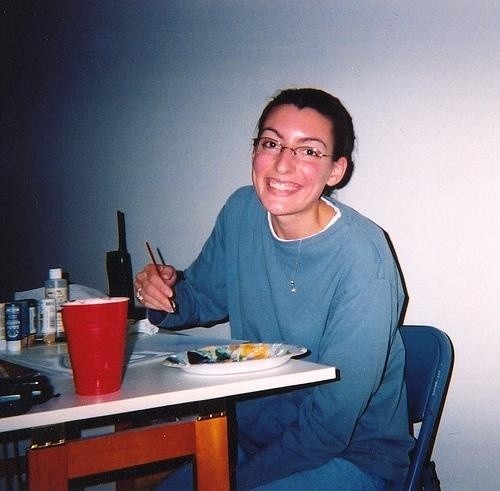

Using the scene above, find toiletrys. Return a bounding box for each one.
[0,302,13,352]
[40,298,58,347]
[4,303,23,354]
[44,267,71,344]
[19,300,39,349]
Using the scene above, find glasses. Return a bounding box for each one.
[250,134,337,163]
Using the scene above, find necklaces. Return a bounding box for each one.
[275,237,306,295]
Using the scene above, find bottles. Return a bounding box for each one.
[0,266,70,355]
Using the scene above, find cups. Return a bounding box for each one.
[59,297,129,396]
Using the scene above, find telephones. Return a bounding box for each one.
[106,210,148,321]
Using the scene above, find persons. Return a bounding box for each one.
[133,86,418,491]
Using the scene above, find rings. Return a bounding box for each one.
[136,288,143,301]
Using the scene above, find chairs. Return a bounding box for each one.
[388,317,454,490]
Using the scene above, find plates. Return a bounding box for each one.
[163,341,313,375]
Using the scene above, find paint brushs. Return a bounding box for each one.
[146,242,177,314]
